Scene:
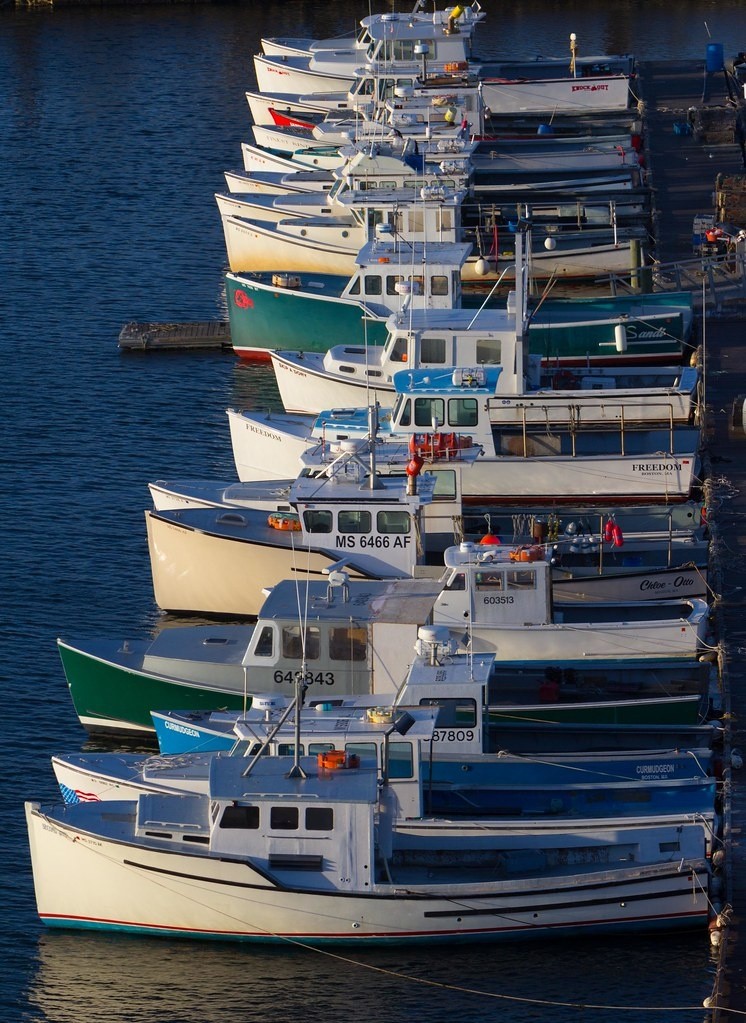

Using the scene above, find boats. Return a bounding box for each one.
[26,348,720,942]
[214,0,746,361]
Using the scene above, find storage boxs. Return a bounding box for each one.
[693,213,714,234]
